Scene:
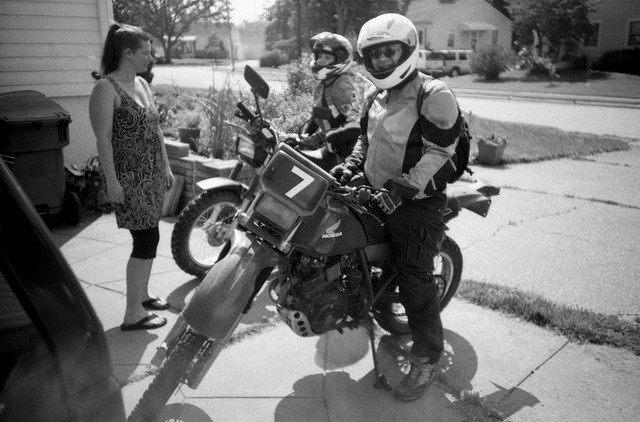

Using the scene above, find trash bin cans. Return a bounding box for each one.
[0,90,83,226]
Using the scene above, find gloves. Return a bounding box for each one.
[281,133,303,151]
[330,157,358,186]
[364,182,402,221]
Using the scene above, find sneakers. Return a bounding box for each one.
[394,353,438,404]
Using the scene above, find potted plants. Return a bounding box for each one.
[477,133,508,165]
[177,112,200,151]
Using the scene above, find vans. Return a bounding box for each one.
[426,50,474,77]
[417,49,430,72]
[0,159,126,421]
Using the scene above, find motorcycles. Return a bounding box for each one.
[170,64,332,278]
[126,141,500,422]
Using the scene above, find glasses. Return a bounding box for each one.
[367,45,398,58]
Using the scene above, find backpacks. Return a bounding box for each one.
[416,78,473,183]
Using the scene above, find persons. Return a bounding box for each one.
[328,13,459,402]
[301,30,377,170]
[87,22,174,331]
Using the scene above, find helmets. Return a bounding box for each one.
[308,28,354,82]
[357,11,422,92]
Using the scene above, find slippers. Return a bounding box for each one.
[119,313,166,330]
[142,296,169,310]
